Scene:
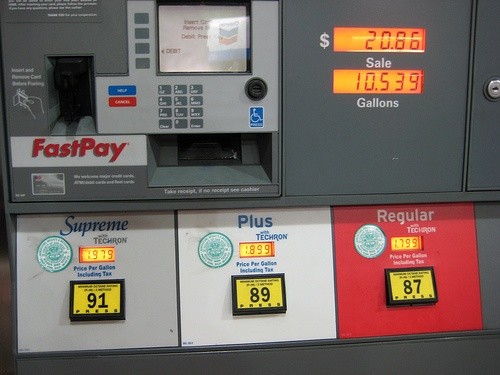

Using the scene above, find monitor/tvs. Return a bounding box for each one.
[154,0,251,74]
